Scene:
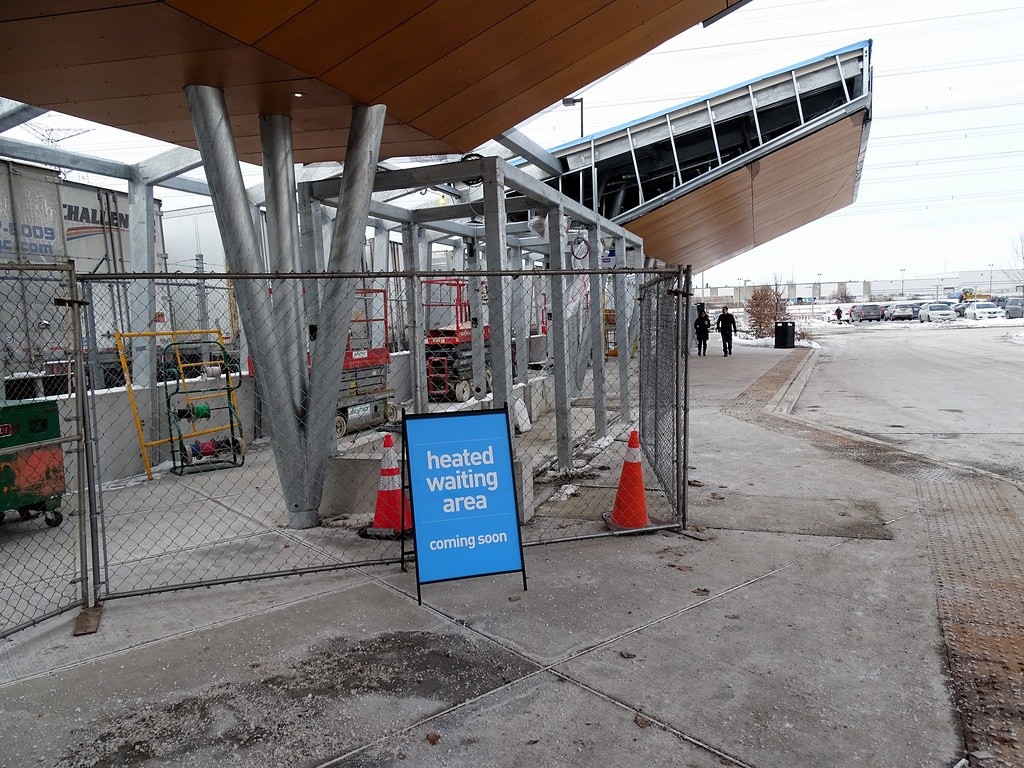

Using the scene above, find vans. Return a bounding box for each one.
[849,304,882,323]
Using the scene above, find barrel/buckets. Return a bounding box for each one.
[4,378,38,400]
[42,375,73,396]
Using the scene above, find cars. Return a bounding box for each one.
[1004,299,1024,319]
[935,294,1011,317]
[822,307,849,322]
[879,305,891,318]
[883,303,914,321]
[964,302,1006,320]
[917,304,958,322]
[910,302,929,318]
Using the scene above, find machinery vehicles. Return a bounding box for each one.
[421,276,523,404]
[242,286,400,440]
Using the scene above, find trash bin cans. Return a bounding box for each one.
[775,321,795,348]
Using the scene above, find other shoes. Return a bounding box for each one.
[697,348,702,356]
[723,348,732,357]
[703,350,706,356]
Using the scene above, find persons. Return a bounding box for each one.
[717,307,736,357]
[835,306,842,320]
[694,310,710,357]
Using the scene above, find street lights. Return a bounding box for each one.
[737,278,743,310]
[988,263,994,294]
[901,269,905,297]
[817,273,822,300]
[562,97,584,139]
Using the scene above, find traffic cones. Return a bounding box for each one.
[357,432,414,540]
[601,431,667,533]
